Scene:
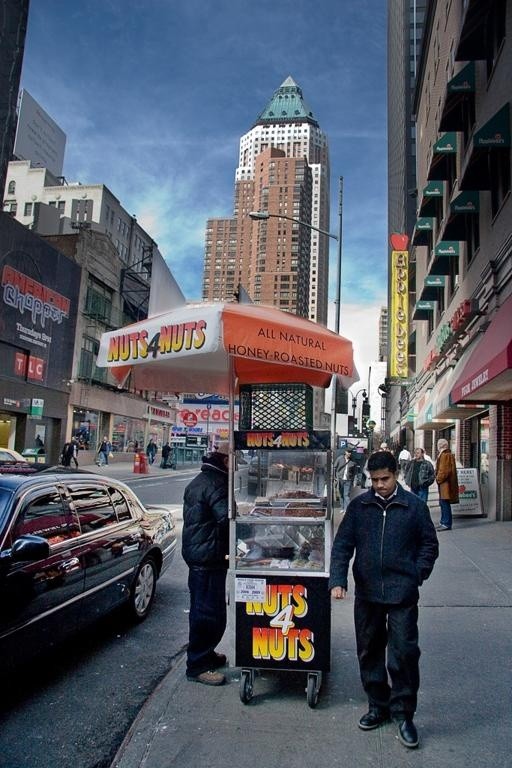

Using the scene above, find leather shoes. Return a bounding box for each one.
[436,525,451,531]
[389,713,420,748]
[358,705,390,730]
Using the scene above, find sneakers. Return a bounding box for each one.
[214,653,227,667]
[188,668,226,686]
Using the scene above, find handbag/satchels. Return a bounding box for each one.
[108,451,113,460]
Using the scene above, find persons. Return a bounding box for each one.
[327,450,440,749]
[33,432,174,471]
[359,441,435,505]
[333,447,357,515]
[433,438,461,533]
[481,453,489,470]
[180,443,248,688]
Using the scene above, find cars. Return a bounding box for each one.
[1,457,179,670]
[0,447,26,461]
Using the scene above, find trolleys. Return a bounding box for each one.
[230,423,337,713]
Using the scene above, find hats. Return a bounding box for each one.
[381,442,389,448]
[211,440,247,465]
[345,448,351,452]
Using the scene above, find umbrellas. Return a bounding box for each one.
[96,295,361,518]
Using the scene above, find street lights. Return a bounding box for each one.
[247,174,346,333]
